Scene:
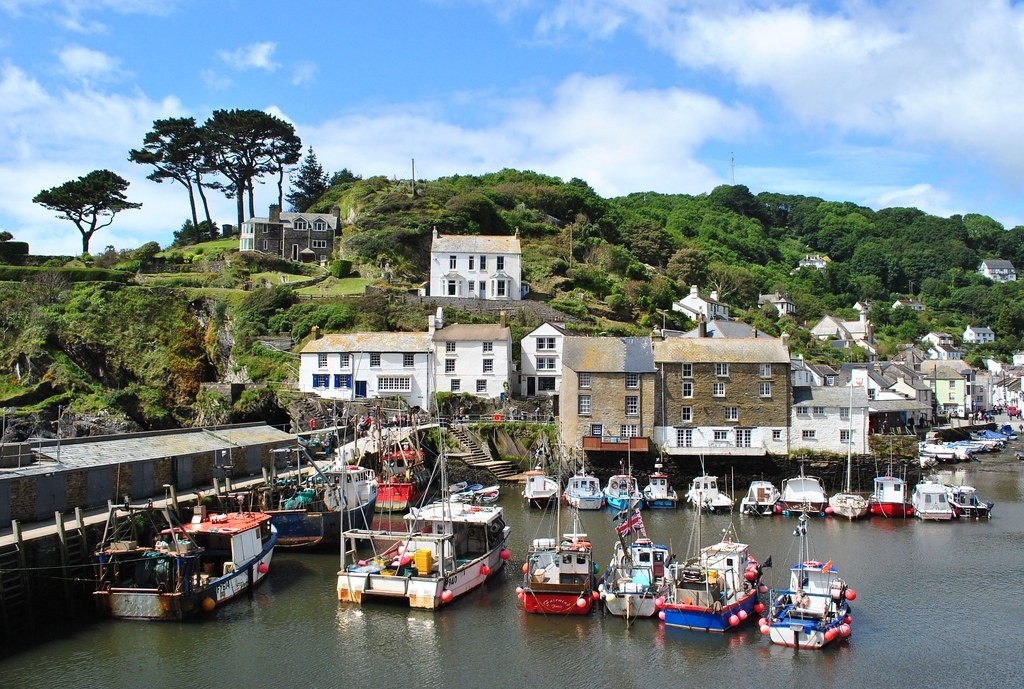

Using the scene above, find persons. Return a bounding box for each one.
[989,411,993,422]
[983,413,988,423]
[459,409,464,424]
[1009,410,1013,420]
[910,414,915,432]
[876,416,905,432]
[156,534,171,553]
[534,407,540,423]
[337,407,422,436]
[920,417,924,430]
[455,408,459,424]
[991,405,1004,415]
[974,410,982,423]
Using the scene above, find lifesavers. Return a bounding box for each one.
[722,541,734,545]
[884,478,893,480]
[572,542,591,547]
[611,481,619,489]
[310,420,315,428]
[803,561,822,566]
[794,594,809,607]
[389,553,411,566]
[340,465,358,470]
[728,544,737,548]
[470,508,483,510]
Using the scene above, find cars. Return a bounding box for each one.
[951,411,957,417]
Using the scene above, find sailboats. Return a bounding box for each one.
[829,380,867,518]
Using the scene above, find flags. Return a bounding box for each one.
[616,512,641,538]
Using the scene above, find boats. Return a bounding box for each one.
[686,475,734,513]
[761,499,856,649]
[911,481,952,521]
[642,471,677,510]
[442,481,466,495]
[451,483,484,500]
[94,486,280,622]
[780,474,829,515]
[356,403,432,512]
[741,481,781,515]
[656,486,768,632]
[598,426,675,621]
[336,392,514,610]
[946,483,994,520]
[522,441,558,508]
[919,426,1019,468]
[514,457,601,615]
[563,450,604,510]
[602,469,643,511]
[264,439,378,550]
[868,476,912,517]
[470,485,499,504]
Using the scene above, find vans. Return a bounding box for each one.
[957,405,971,419]
[1007,404,1021,416]
